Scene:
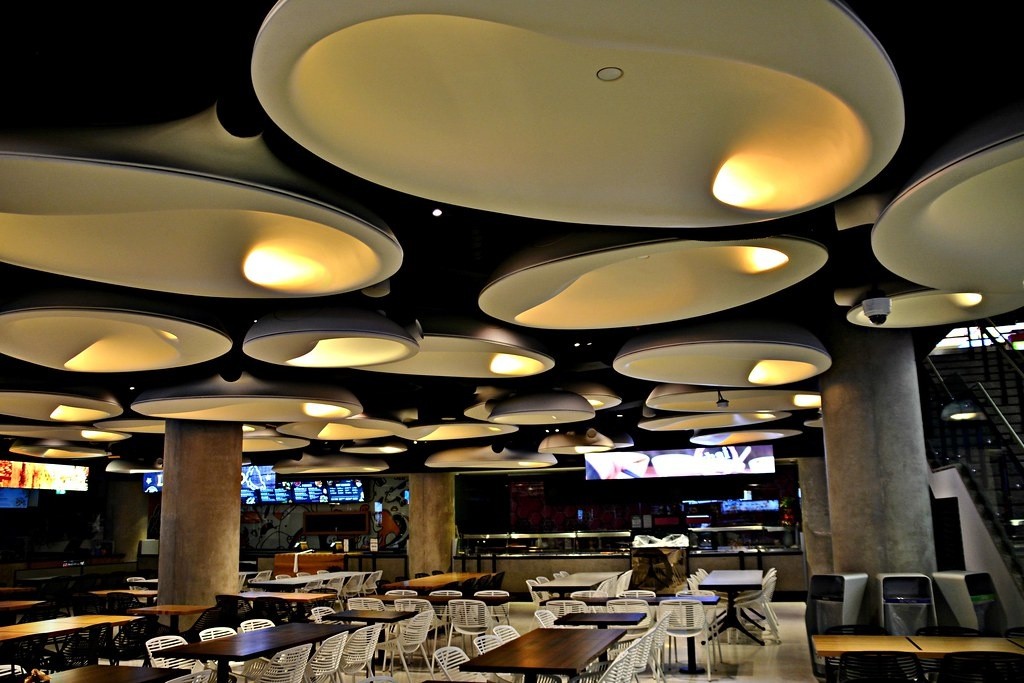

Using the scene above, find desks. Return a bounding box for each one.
[90,590,158,603]
[531,571,624,591]
[45,664,191,683]
[0,616,141,665]
[321,609,419,683]
[457,628,629,683]
[699,570,768,645]
[128,605,215,633]
[553,612,648,670]
[0,601,46,624]
[240,592,333,611]
[382,572,496,592]
[542,597,721,675]
[246,571,374,590]
[811,635,1024,683]
[151,624,367,683]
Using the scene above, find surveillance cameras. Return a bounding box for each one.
[862,297,892,325]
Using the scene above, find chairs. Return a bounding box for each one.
[0,568,780,683]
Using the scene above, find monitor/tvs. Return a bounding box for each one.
[141,472,163,496]
[0,487,30,509]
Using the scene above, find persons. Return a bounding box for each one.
[781,506,795,527]
[665,504,697,516]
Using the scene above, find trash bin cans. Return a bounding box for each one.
[933,569,1007,635]
[874,572,938,636]
[804,572,870,682]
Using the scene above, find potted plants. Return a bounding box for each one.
[779,496,796,522]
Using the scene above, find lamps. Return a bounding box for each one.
[0,0,1024,483]
